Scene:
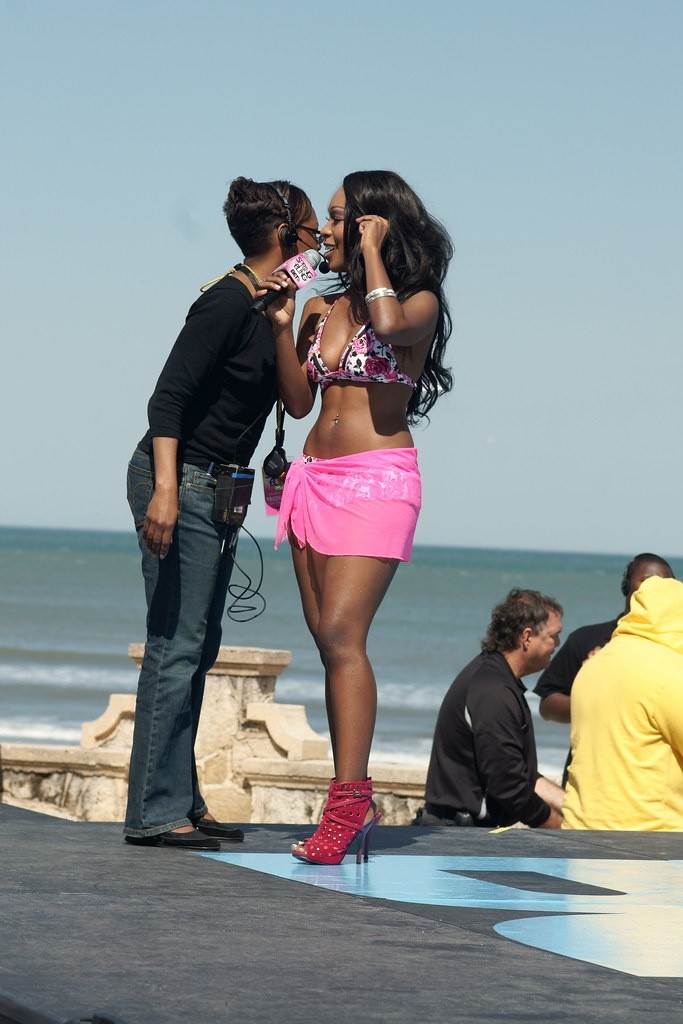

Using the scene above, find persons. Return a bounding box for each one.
[123,176,321,849]
[412,549,683,833]
[253,170,455,868]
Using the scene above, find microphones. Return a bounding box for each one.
[249,249,321,316]
[298,238,331,274]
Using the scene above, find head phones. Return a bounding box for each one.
[266,182,298,246]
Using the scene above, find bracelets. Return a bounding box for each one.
[364,287,397,305]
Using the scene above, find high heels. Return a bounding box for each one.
[292,777,383,865]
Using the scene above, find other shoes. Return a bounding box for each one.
[124,829,220,850]
[190,818,244,843]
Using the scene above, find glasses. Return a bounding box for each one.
[277,221,325,245]
